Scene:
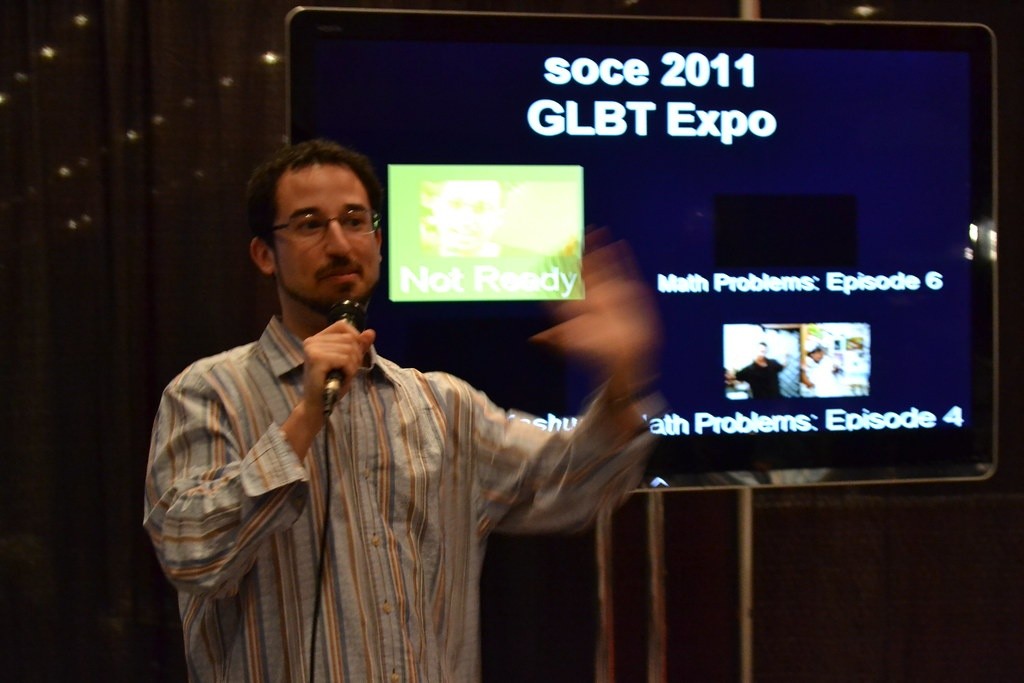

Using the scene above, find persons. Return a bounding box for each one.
[801,341,849,397]
[144,141,664,683]
[722,342,793,399]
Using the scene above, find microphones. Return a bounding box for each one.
[322,301,365,416]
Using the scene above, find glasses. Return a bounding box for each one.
[273,208,382,239]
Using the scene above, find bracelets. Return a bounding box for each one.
[613,383,665,405]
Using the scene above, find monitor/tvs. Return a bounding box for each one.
[286,5,999,489]
